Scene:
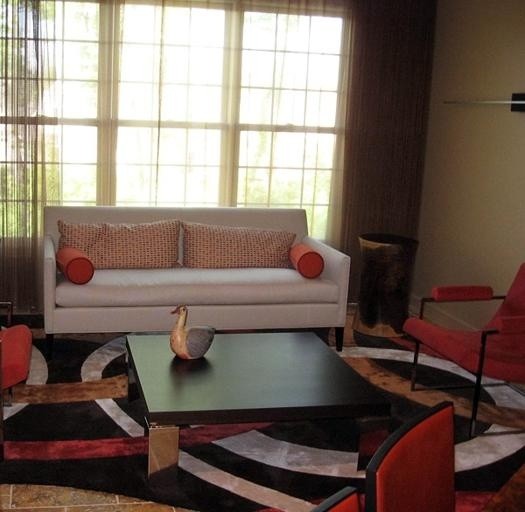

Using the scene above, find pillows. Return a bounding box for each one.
[57,219,181,269]
[290,244,324,278]
[180,220,296,269]
[55,247,94,283]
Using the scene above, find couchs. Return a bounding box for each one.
[42,205,351,351]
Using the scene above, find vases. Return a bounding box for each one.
[353,234,419,346]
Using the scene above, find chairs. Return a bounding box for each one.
[313,401,456,512]
[404,264,524,437]
[0,302,32,406]
